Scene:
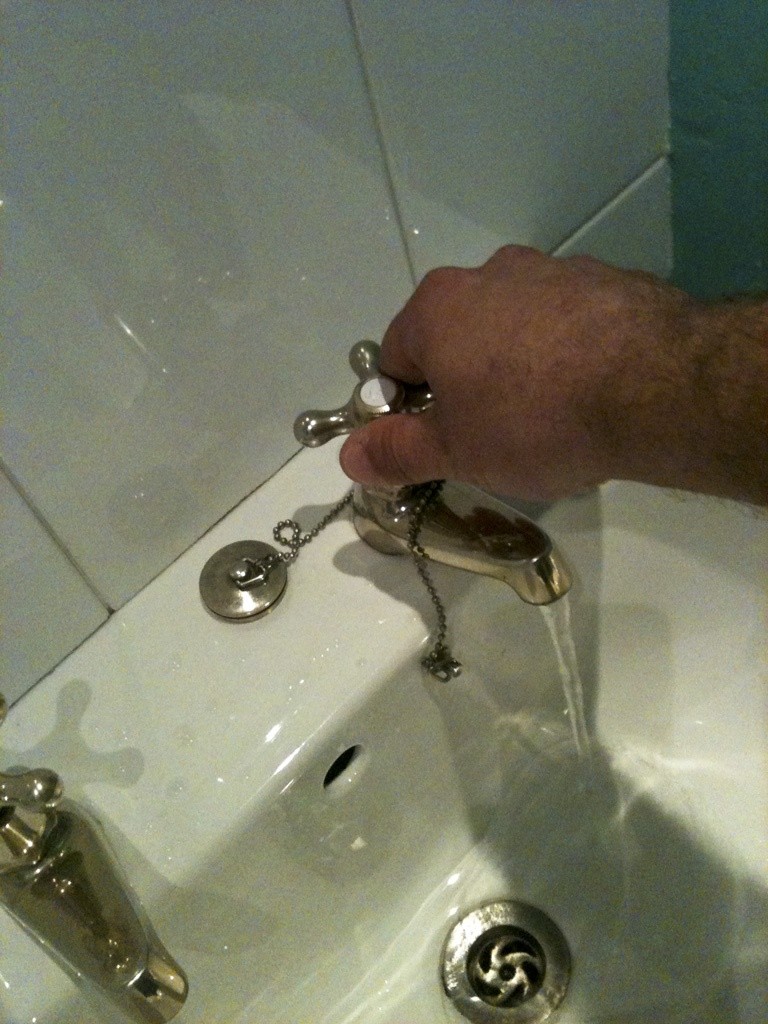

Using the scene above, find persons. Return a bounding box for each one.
[341,243,768,506]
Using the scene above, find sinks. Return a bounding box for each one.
[37,521,768,1022]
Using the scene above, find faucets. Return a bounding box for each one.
[0,755,194,1024]
[289,338,577,609]
